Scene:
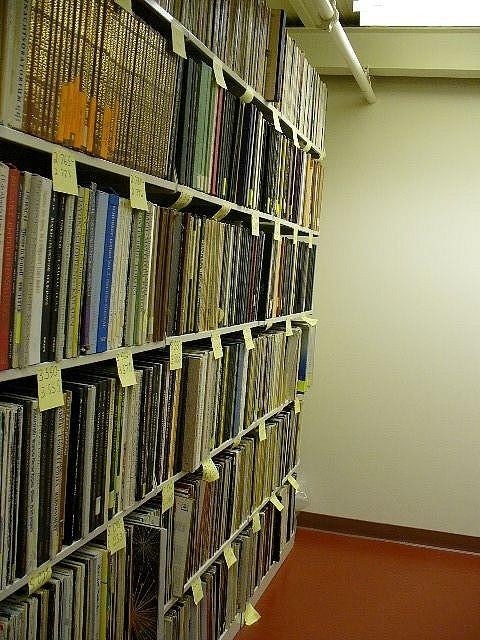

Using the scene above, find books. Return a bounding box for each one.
[0,1,328,233]
[1,319,318,640]
[1,161,317,370]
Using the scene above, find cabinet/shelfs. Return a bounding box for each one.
[4,1,326,639]
